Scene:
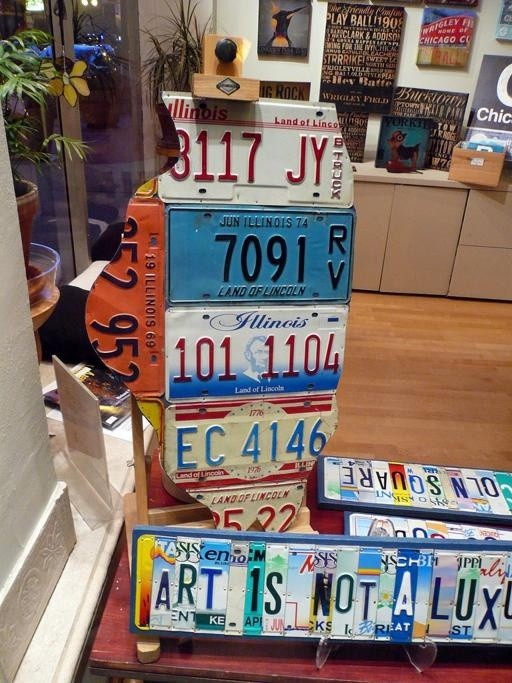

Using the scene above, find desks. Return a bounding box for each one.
[89,457,512,683]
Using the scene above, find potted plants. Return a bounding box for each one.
[0,27,97,281]
[138,1,215,145]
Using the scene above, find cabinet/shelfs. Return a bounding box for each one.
[349,178,512,302]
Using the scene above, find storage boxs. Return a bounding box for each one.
[447,141,507,188]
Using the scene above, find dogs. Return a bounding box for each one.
[387,137,421,168]
